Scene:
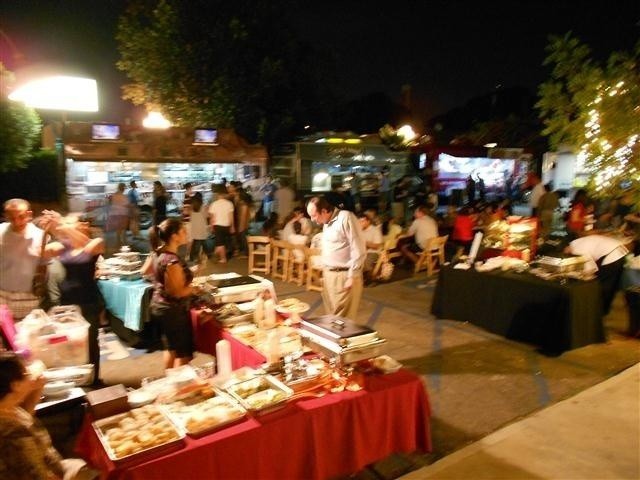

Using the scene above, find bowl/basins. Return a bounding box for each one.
[275,302,311,318]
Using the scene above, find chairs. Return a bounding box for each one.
[243,219,450,295]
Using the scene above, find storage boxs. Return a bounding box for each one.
[19,312,91,372]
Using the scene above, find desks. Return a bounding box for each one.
[189,274,346,365]
[432,257,608,359]
[93,265,154,328]
[83,354,423,478]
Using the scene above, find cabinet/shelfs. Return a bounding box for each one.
[479,213,540,264]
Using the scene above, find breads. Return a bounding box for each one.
[183,397,244,434]
[90,404,183,462]
[231,375,288,409]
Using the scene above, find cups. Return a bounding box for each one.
[252,298,277,327]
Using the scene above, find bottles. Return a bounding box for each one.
[215,340,232,384]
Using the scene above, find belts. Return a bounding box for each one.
[328,266,350,272]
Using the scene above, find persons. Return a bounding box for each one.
[307,196,362,319]
[0,198,107,380]
[112,176,278,266]
[0,352,66,480]
[142,217,203,366]
[263,206,315,262]
[450,175,640,245]
[330,166,439,276]
[561,233,629,317]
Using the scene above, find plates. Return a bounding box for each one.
[127,391,159,407]
[43,382,75,398]
[369,355,402,374]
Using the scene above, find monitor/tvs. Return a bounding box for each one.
[88,122,122,143]
[192,128,219,146]
